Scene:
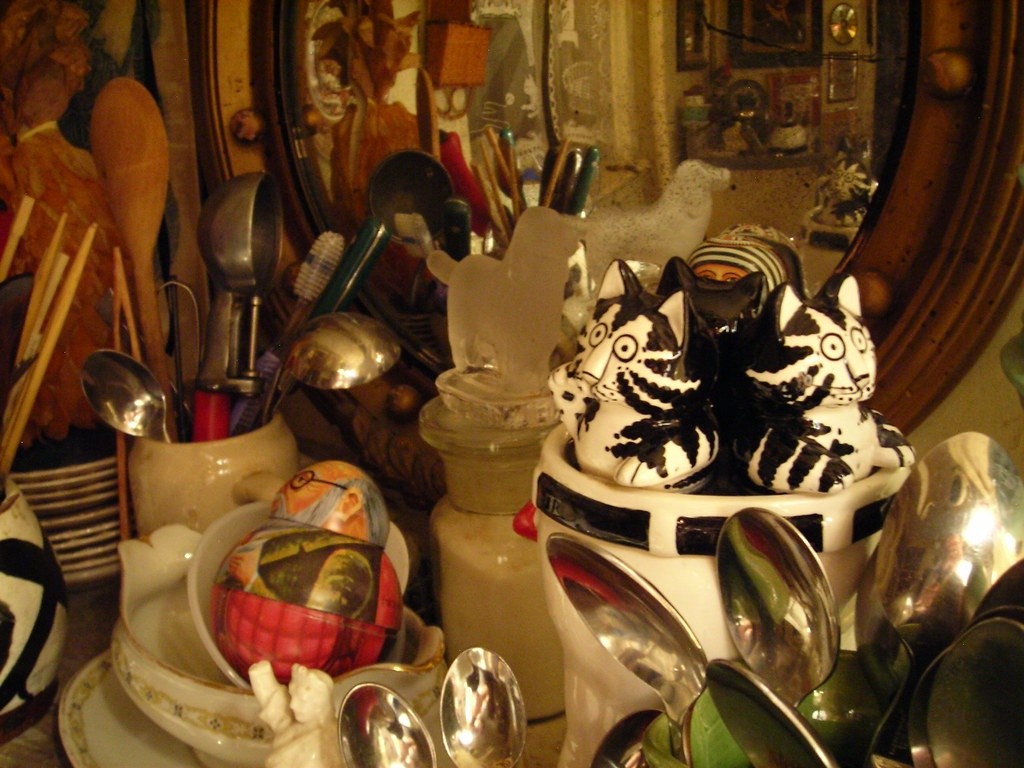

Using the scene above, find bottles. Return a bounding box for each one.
[0,478,67,718]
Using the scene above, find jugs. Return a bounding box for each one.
[768,101,807,152]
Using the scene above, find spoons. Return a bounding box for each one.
[337,682,437,768]
[545,533,708,768]
[80,350,173,445]
[708,659,840,768]
[850,429,1024,768]
[926,620,1024,768]
[716,506,841,708]
[440,647,527,768]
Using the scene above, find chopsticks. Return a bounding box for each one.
[0,198,98,489]
[470,126,568,252]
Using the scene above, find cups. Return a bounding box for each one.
[640,648,908,768]
[686,95,704,105]
[127,407,301,536]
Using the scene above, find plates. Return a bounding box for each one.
[11,451,124,587]
[57,650,205,768]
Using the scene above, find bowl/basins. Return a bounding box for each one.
[187,497,410,689]
[112,522,447,768]
